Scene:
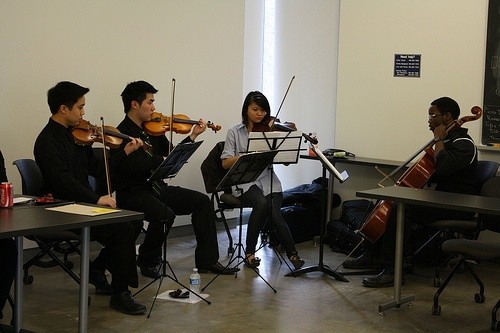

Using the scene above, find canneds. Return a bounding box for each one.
[0,182,13,207]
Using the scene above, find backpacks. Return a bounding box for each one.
[327,219,364,258]
[406,222,475,269]
[339,198,376,228]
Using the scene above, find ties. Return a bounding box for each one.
[140,130,163,197]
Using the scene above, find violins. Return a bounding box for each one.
[71,118,153,151]
[143,111,221,138]
[252,116,319,145]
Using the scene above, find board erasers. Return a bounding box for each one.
[487,143,500,147]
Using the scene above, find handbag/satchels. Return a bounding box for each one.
[282,183,325,237]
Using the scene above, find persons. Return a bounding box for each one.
[220,90,306,269]
[0,150,17,319]
[34,82,147,315]
[112,80,240,280]
[342,96,479,288]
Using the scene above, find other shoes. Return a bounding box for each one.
[342,251,383,269]
[79,261,112,291]
[362,266,406,287]
[109,289,146,314]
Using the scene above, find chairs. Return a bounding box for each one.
[399,160,500,313]
[201,142,275,258]
[12,159,82,284]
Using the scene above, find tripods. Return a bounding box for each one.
[131,140,212,320]
[231,132,304,278]
[201,149,278,293]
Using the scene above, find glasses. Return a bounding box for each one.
[429,112,448,120]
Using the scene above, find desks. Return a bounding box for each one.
[0,187,144,333]
[355,187,500,313]
[285,154,415,245]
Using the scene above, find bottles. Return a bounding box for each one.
[309,132,318,156]
[190,268,200,302]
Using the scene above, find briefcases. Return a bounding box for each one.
[276,202,316,247]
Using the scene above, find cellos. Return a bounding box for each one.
[333,106,483,271]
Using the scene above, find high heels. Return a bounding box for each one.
[290,254,306,269]
[243,253,261,267]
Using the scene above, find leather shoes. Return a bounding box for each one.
[136,255,160,279]
[196,260,240,274]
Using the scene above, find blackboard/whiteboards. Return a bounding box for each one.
[480,0,500,151]
